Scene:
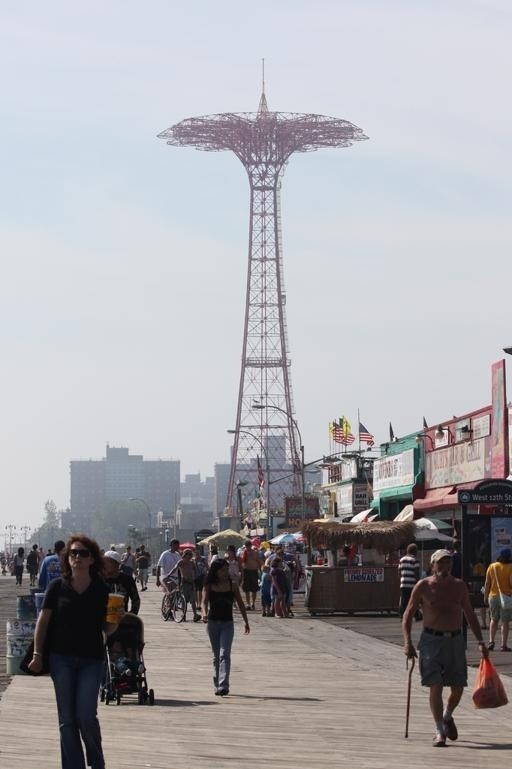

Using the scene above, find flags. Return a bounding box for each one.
[329,416,374,447]
[256,456,265,489]
[423,417,427,429]
[390,423,395,442]
[295,451,300,469]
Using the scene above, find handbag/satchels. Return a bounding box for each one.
[500,592,512,611]
[19,640,51,675]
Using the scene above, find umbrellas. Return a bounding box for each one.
[196,528,250,547]
[266,531,306,545]
[411,517,457,579]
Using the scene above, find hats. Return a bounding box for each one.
[430,549,454,565]
[498,545,512,557]
[224,545,238,553]
[103,550,122,565]
[181,548,192,558]
[168,538,179,546]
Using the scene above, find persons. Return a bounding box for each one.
[121,541,296,619]
[202,558,250,695]
[401,549,489,747]
[484,548,512,650]
[27,533,125,768]
[98,546,148,698]
[13,540,68,609]
[398,544,423,622]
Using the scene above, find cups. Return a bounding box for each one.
[105,592,125,625]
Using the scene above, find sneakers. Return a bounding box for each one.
[432,716,458,747]
[487,641,512,651]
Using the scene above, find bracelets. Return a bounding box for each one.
[34,651,44,657]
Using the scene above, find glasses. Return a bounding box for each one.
[66,548,91,558]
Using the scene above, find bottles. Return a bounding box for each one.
[317,555,325,565]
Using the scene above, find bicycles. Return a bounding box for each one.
[157,576,191,625]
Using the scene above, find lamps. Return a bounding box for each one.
[434,422,454,444]
[415,433,434,450]
[454,424,474,433]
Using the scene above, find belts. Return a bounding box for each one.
[423,627,461,639]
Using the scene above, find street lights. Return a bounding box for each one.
[127,496,153,549]
[250,403,306,524]
[158,520,170,544]
[20,525,32,554]
[226,429,272,538]
[127,524,139,533]
[4,523,17,555]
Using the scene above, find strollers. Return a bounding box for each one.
[99,609,156,705]
